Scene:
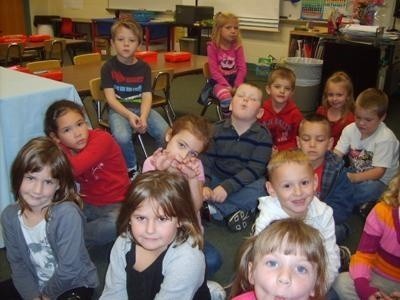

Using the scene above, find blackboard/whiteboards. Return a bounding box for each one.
[105,0,397,33]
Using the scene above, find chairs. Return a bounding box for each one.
[201,61,222,119]
[28,58,61,69]
[73,53,102,64]
[7,42,23,64]
[90,20,109,52]
[89,76,147,158]
[60,18,87,40]
[44,39,66,64]
[149,67,176,127]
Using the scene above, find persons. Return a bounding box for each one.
[0,137,102,300]
[142,112,222,280]
[100,18,171,184]
[97,170,226,300]
[199,68,399,300]
[207,11,248,122]
[42,99,129,249]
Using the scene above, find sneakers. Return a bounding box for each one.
[128,164,142,183]
[200,201,210,222]
[205,281,226,300]
[225,210,254,232]
[360,202,374,217]
[341,246,351,265]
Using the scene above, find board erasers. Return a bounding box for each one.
[280,16,288,19]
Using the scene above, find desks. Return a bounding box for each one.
[34,15,175,55]
[28,51,209,101]
[0,33,86,66]
[288,25,400,93]
[1,66,92,247]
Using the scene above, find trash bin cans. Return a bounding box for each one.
[283,57,323,112]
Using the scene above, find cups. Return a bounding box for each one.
[328,20,340,34]
